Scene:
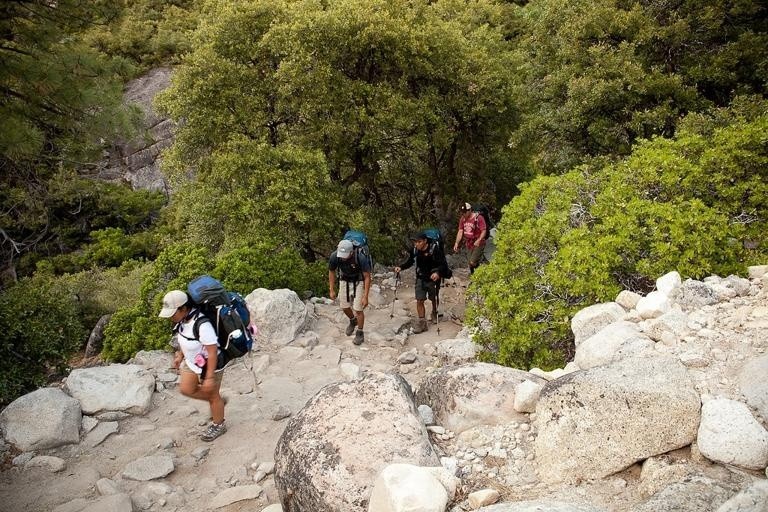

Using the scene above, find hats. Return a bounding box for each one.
[336,240,353,259]
[410,232,426,240]
[460,203,472,214]
[158,290,188,318]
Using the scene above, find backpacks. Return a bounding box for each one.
[171,275,254,371]
[335,231,373,281]
[463,202,491,240]
[412,229,452,280]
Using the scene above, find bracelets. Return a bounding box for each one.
[205,375,216,380]
[478,237,482,241]
[455,239,460,243]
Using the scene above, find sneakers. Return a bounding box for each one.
[346,317,357,336]
[414,320,428,334]
[431,310,437,324]
[200,419,227,443]
[353,330,364,345]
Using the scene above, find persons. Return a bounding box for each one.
[158,290,230,442]
[327,239,371,345]
[394,232,448,334]
[453,202,488,277]
